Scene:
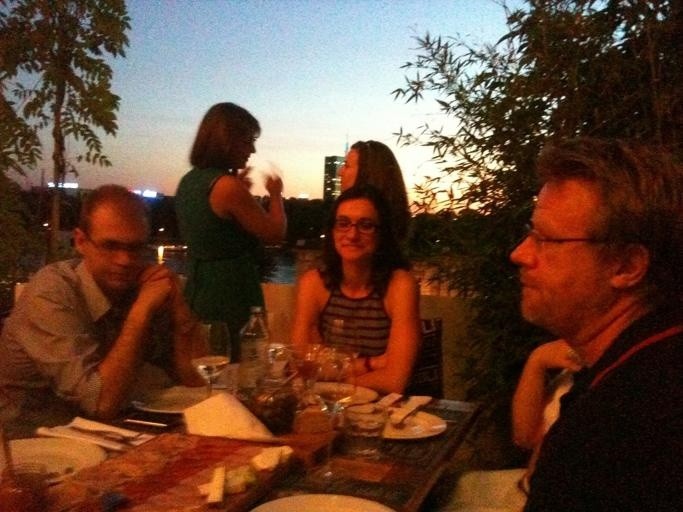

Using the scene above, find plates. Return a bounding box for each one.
[248,494,397,512]
[131,383,227,414]
[310,381,448,440]
[0,437,108,484]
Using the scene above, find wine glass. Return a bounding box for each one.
[283,318,363,414]
[187,321,232,399]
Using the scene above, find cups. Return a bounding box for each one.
[343,404,386,468]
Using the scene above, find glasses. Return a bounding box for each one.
[525,224,606,255]
[244,136,256,144]
[333,220,383,234]
[86,237,148,255]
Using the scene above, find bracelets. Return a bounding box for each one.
[363,357,373,372]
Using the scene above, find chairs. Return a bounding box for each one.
[409,320,446,396]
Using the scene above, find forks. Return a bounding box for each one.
[70,424,146,442]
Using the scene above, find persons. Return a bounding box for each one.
[0,186,212,440]
[511,139,682,511]
[174,102,287,363]
[442,339,582,511]
[290,185,421,398]
[338,139,414,239]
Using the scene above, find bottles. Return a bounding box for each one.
[238,307,270,402]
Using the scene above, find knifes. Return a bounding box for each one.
[68,425,137,448]
